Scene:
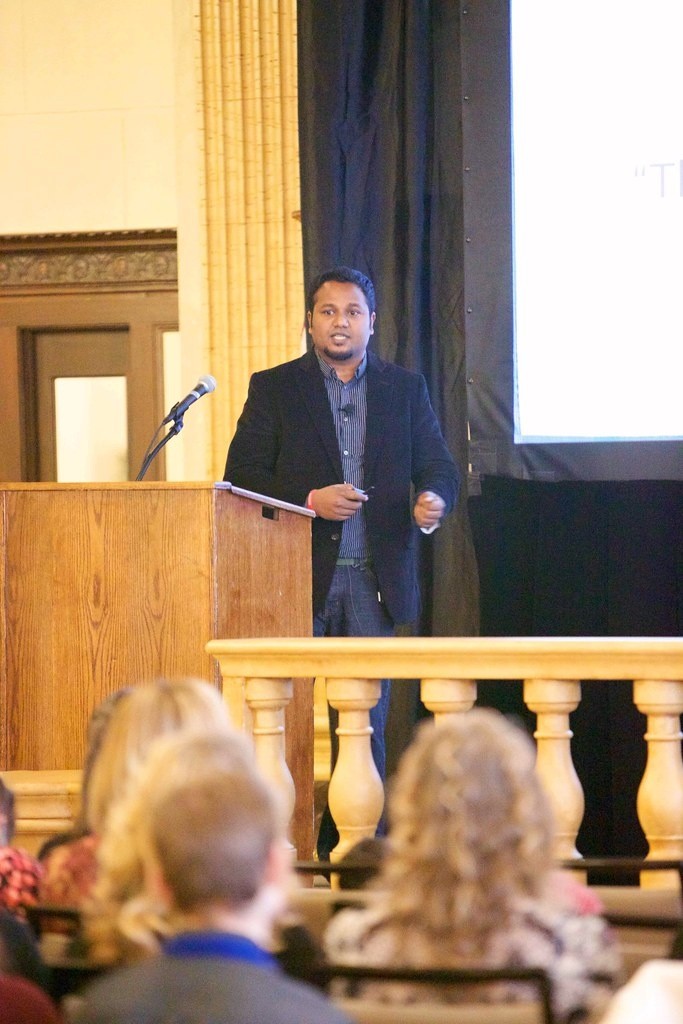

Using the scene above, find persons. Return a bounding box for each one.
[0,672,246,1024]
[319,705,622,1024]
[222,266,459,891]
[70,739,352,1024]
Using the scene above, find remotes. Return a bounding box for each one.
[346,485,375,502]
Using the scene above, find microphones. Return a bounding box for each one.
[160,374,217,425]
[344,404,355,414]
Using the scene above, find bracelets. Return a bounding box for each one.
[307,490,316,509]
